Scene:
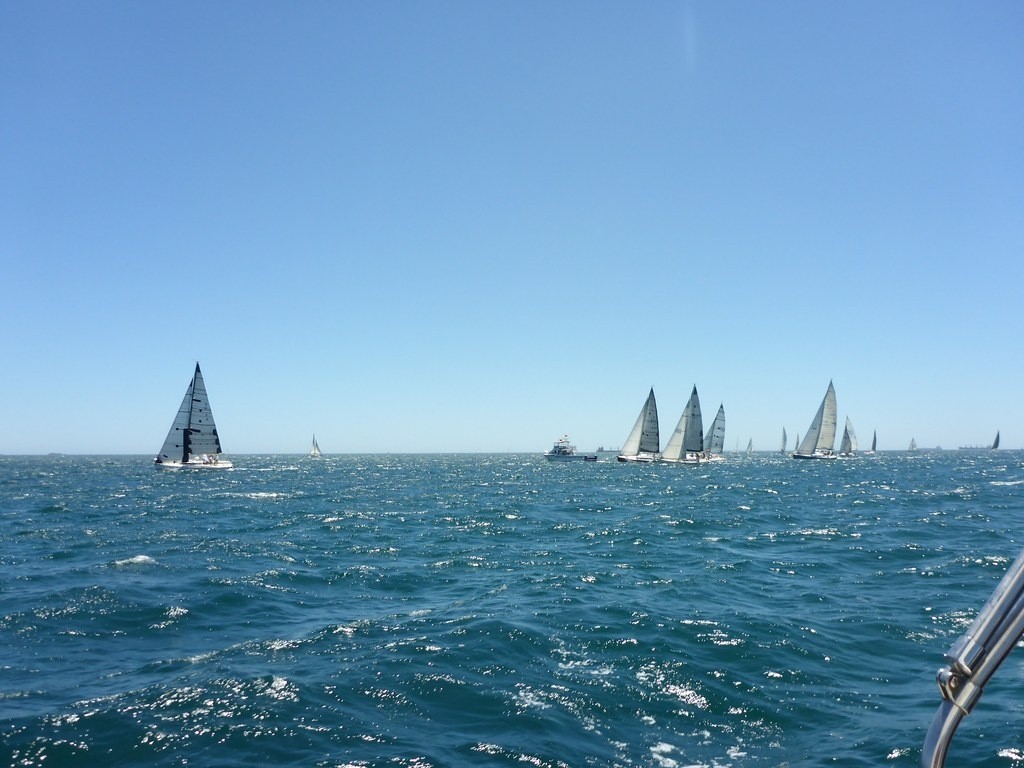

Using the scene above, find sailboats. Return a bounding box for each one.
[742,437,755,456]
[616,384,661,463]
[779,425,787,456]
[792,377,839,460]
[794,432,800,450]
[702,399,728,461]
[907,436,917,453]
[992,428,1001,450]
[308,433,322,458]
[863,428,876,453]
[659,383,709,465]
[839,414,857,457]
[153,360,233,471]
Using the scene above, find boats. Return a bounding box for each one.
[542,434,599,463]
